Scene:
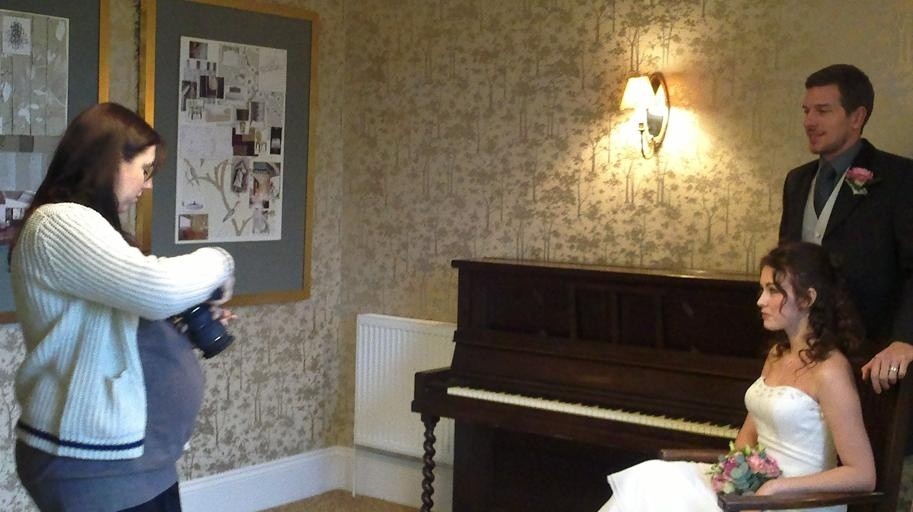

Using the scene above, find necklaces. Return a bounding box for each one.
[782,351,809,369]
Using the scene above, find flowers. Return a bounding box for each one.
[844,166,875,193]
[705,439,781,496]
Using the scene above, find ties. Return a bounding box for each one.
[813,164,834,219]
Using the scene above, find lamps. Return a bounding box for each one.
[617,72,668,159]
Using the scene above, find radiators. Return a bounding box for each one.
[349,312,458,472]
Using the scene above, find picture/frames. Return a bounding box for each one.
[1,0,112,327]
[135,3,314,305]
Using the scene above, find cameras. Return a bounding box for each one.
[186,303,235,359]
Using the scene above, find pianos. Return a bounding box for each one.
[410,256,789,512]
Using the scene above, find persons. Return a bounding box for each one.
[776,61,912,396]
[594,240,876,511]
[7,100,240,512]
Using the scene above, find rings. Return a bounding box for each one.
[889,367,898,373]
